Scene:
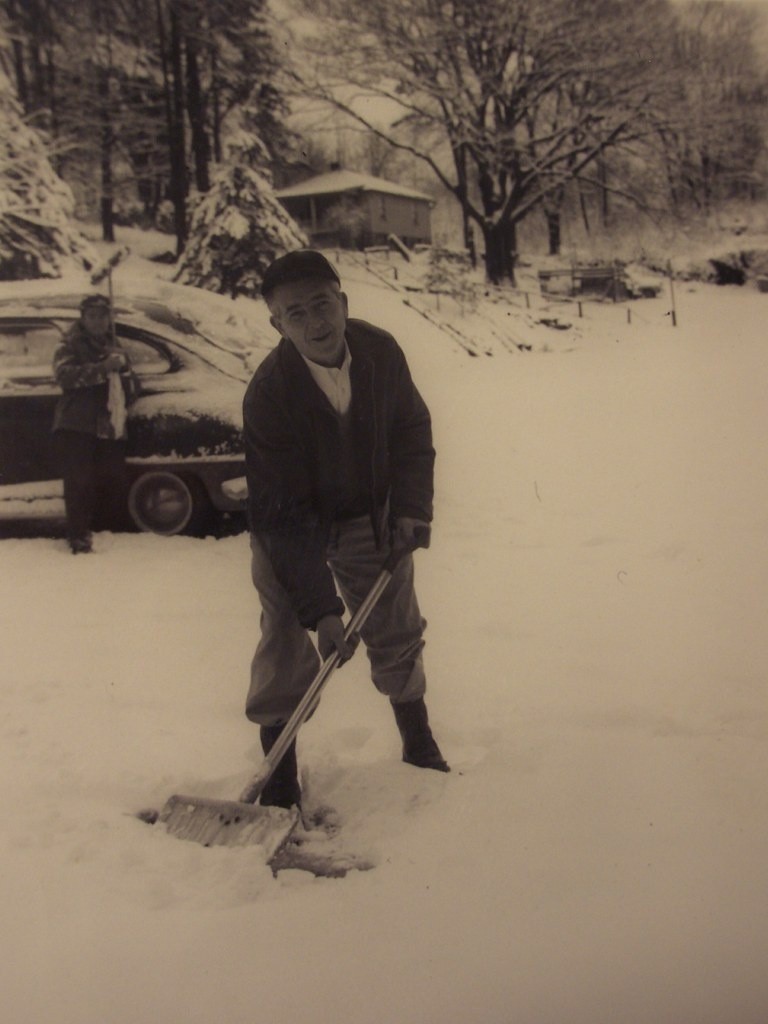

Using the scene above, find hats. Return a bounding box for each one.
[257,250,342,296]
[81,294,112,308]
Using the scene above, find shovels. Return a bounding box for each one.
[153,525,432,867]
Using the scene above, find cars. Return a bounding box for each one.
[581,263,662,299]
[1,275,281,539]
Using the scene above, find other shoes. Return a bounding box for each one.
[73,540,91,554]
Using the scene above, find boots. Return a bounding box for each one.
[389,700,451,773]
[259,727,302,810]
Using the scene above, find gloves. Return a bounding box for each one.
[378,517,430,553]
[318,614,361,668]
[104,353,126,371]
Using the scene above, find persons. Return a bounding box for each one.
[52,291,139,556]
[242,246,452,814]
